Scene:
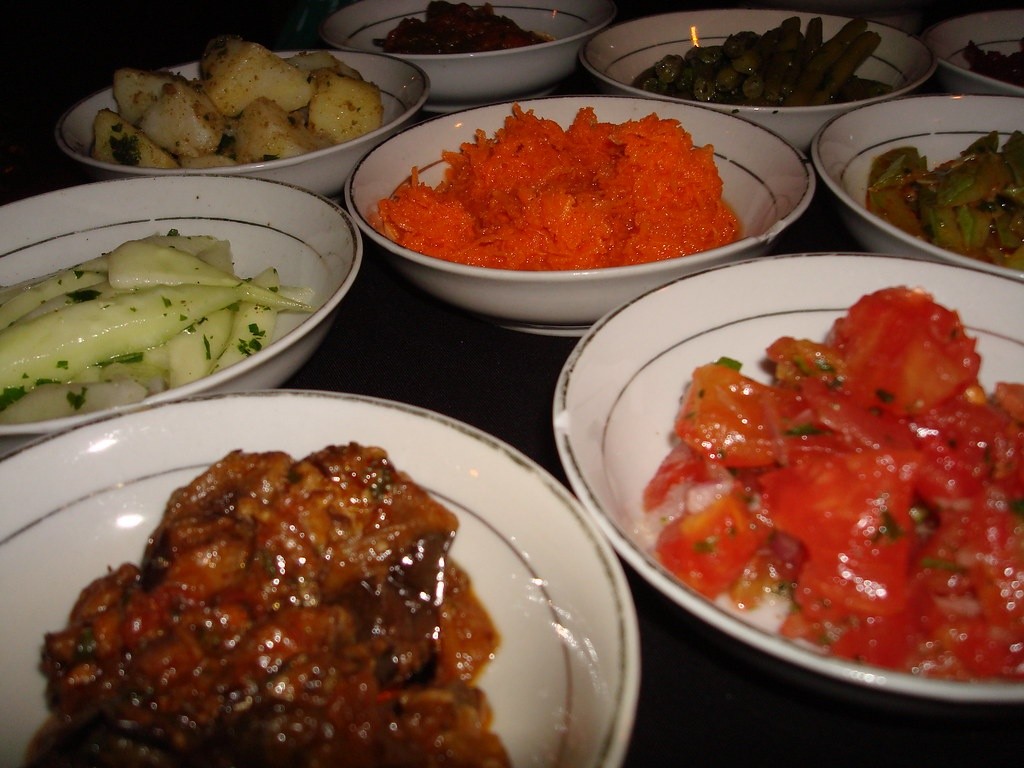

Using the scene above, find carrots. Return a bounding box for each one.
[376,103,740,271]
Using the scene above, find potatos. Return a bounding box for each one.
[88,35,383,169]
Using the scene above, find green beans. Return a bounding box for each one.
[635,16,882,108]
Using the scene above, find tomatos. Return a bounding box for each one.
[640,288,1024,684]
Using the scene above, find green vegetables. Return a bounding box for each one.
[0,227,281,412]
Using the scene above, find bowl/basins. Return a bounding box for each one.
[809,95,1024,280]
[575,6,937,149]
[342,94,817,338]
[929,10,1024,94]
[553,250,1024,704]
[0,389,643,768]
[52,46,429,198]
[318,0,614,113]
[0,175,365,430]
